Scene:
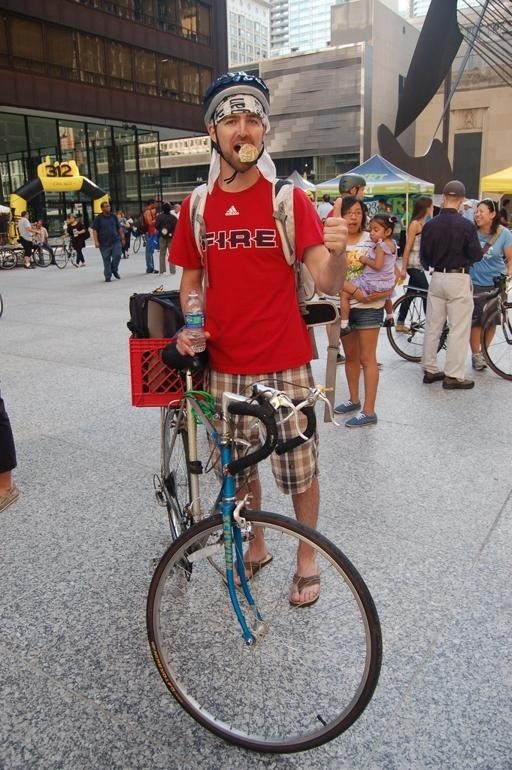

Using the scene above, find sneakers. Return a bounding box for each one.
[81,262,86,267]
[423,371,445,383]
[333,400,361,414]
[472,353,488,371]
[73,262,79,267]
[147,269,158,274]
[344,411,377,428]
[336,355,345,364]
[0,485,20,512]
[443,376,474,389]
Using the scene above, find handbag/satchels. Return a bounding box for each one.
[127,284,186,392]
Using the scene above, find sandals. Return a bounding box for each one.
[288,574,321,608]
[223,550,272,585]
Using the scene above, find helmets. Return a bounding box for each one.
[213,93,264,125]
[339,174,366,193]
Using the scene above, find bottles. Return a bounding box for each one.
[184,289,205,353]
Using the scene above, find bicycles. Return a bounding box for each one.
[133,224,147,253]
[0,233,54,270]
[54,236,77,269]
[146,342,384,755]
[387,275,511,380]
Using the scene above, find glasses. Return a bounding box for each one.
[345,209,362,217]
[484,198,495,210]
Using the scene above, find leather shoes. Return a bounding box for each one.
[113,272,120,279]
[25,264,35,269]
[106,278,111,282]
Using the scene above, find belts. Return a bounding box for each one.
[434,266,469,273]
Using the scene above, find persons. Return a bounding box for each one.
[1,398,21,517]
[116,209,132,260]
[339,214,398,338]
[467,198,512,372]
[462,201,476,224]
[326,174,366,218]
[417,181,483,390]
[59,212,89,268]
[91,201,126,283]
[169,72,350,608]
[32,220,57,267]
[170,203,181,219]
[155,203,177,275]
[325,196,387,428]
[395,196,435,334]
[141,199,159,274]
[17,210,38,269]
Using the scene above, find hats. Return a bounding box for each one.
[443,180,465,196]
[202,73,271,126]
[463,201,473,207]
